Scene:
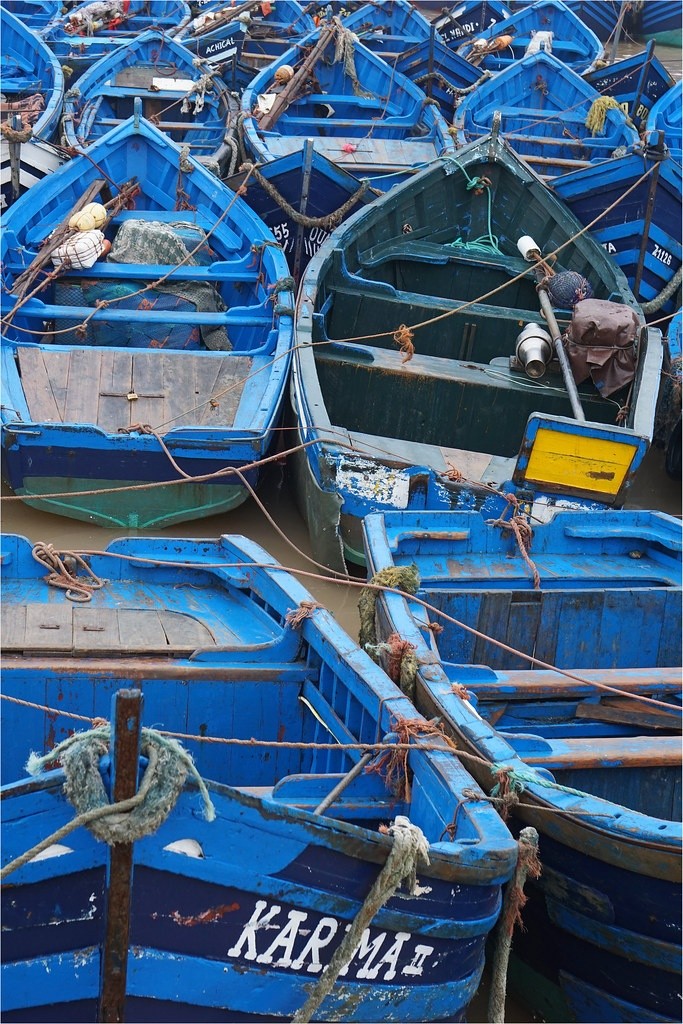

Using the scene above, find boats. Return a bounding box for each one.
[0,93,297,536]
[0,4,65,147]
[240,4,456,198]
[0,531,520,1024]
[642,79,681,172]
[359,504,682,1024]
[60,19,239,181]
[1,1,682,82]
[287,108,664,586]
[450,40,643,184]
